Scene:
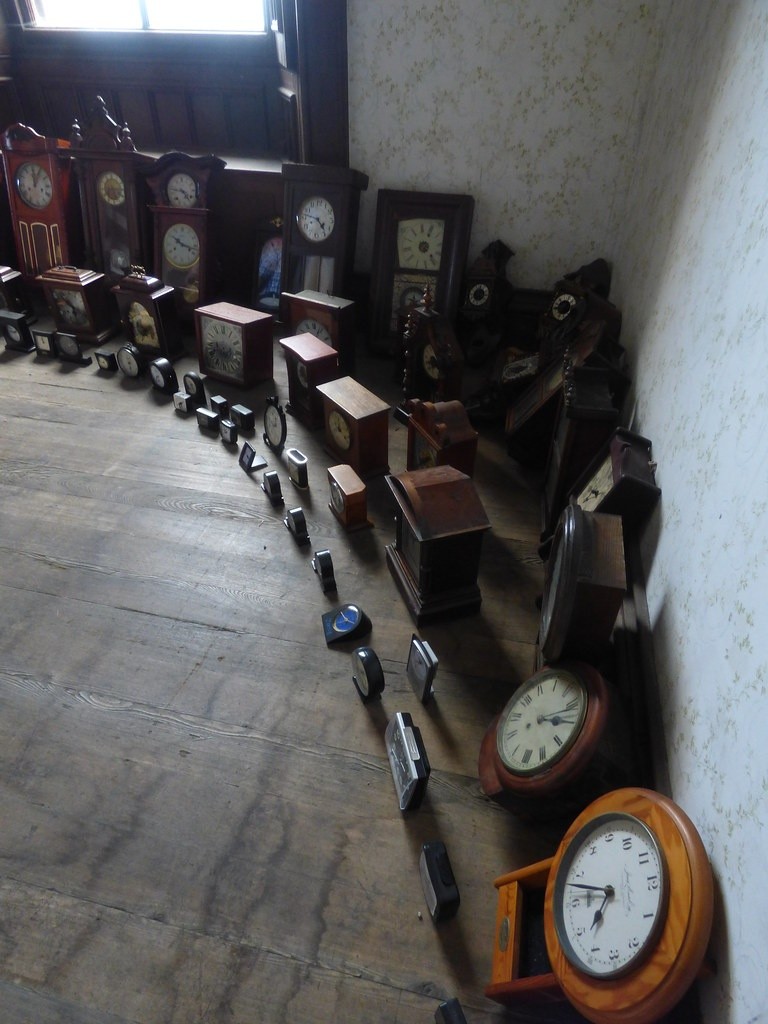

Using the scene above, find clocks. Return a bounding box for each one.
[361,188,631,476]
[278,330,344,433]
[486,787,714,1024]
[238,443,339,596]
[349,646,387,705]
[191,302,275,388]
[406,631,441,704]
[384,712,433,813]
[279,286,357,379]
[327,464,375,534]
[0,91,261,442]
[276,160,371,300]
[317,375,395,486]
[546,428,659,539]
[262,394,289,457]
[478,661,613,799]
[534,491,634,662]
[321,601,374,650]
[381,463,493,628]
[418,839,462,926]
